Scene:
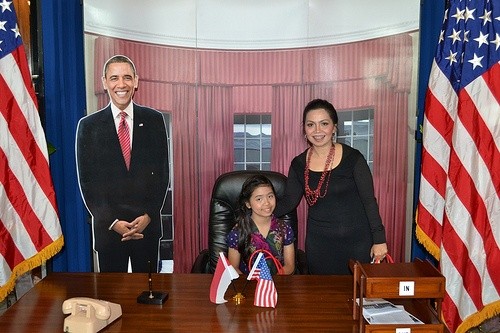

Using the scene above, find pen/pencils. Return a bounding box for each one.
[147,260,154,297]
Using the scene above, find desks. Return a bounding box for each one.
[0,271,451,333]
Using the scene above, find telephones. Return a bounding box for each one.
[63,296,121,333]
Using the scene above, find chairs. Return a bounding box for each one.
[191,170,309,275]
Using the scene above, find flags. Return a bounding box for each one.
[212,303,239,333]
[247,252,278,308]
[248,309,277,333]
[0,0,64,303]
[416,1,500,333]
[209,252,239,304]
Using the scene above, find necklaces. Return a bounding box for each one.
[304,143,335,205]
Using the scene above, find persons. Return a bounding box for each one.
[274,98,388,274]
[224,174,296,275]
[74,54,171,273]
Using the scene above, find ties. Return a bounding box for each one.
[117,112,130,171]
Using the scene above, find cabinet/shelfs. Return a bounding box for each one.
[348,257,446,333]
